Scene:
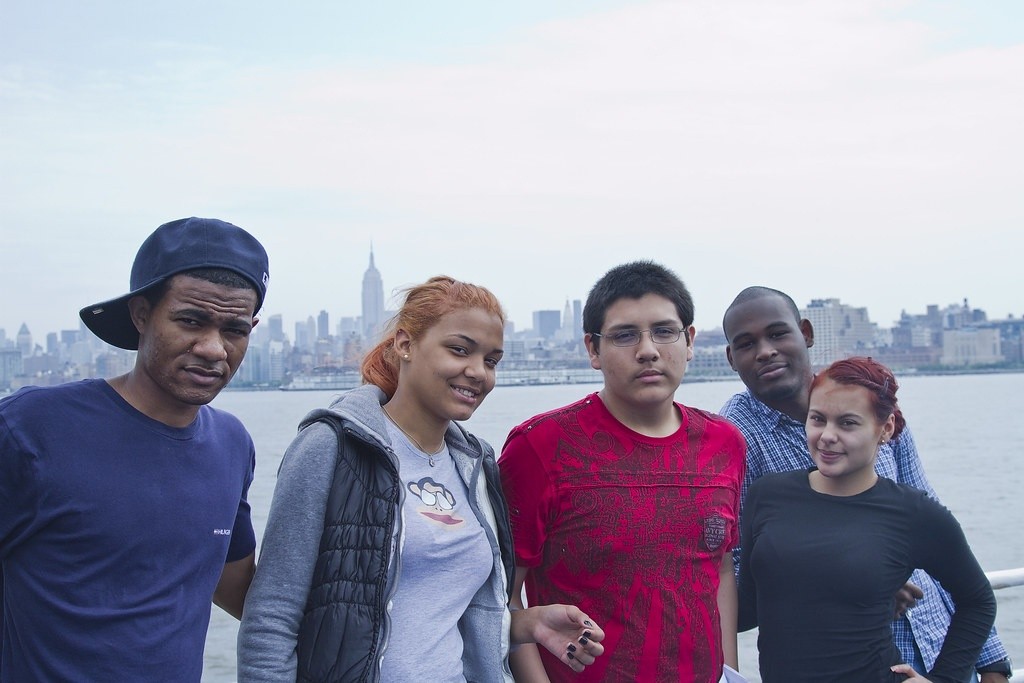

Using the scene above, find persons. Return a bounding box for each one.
[0,214,269,683]
[719,288,1012,683]
[238,276,604,683]
[497,260,746,683]
[733,355,996,683]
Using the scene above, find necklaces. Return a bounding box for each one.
[382,404,445,467]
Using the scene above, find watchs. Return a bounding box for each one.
[978,657,1012,679]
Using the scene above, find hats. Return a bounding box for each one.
[79,216,269,350]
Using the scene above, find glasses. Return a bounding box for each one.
[590,328,688,347]
